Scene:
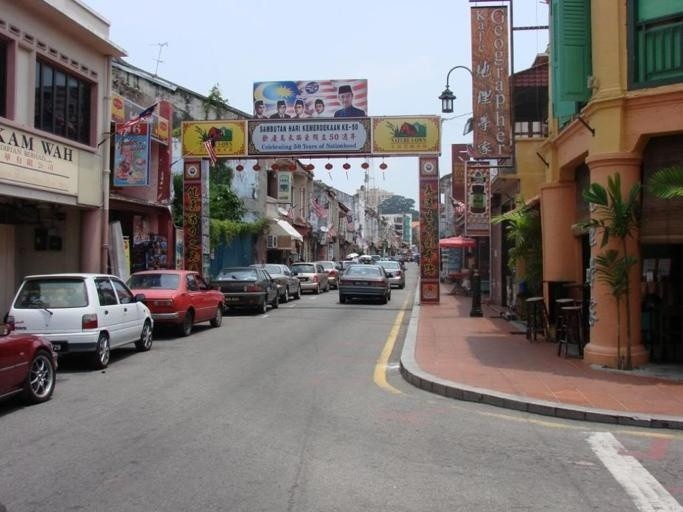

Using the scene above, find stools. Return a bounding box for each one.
[525,297,585,358]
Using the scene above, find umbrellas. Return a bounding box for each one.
[439,235,477,272]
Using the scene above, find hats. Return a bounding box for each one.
[277,101,285,105]
[315,99,322,104]
[255,101,263,105]
[339,85,351,93]
[296,100,303,104]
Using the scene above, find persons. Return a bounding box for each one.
[254,86,365,118]
[467,252,475,273]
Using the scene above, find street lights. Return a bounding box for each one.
[439,65,472,113]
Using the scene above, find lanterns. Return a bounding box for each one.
[233,158,388,184]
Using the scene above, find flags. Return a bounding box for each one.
[116,103,156,137]
[346,215,353,231]
[311,198,328,219]
[327,227,336,238]
[451,199,466,217]
[287,208,294,220]
[204,137,218,167]
